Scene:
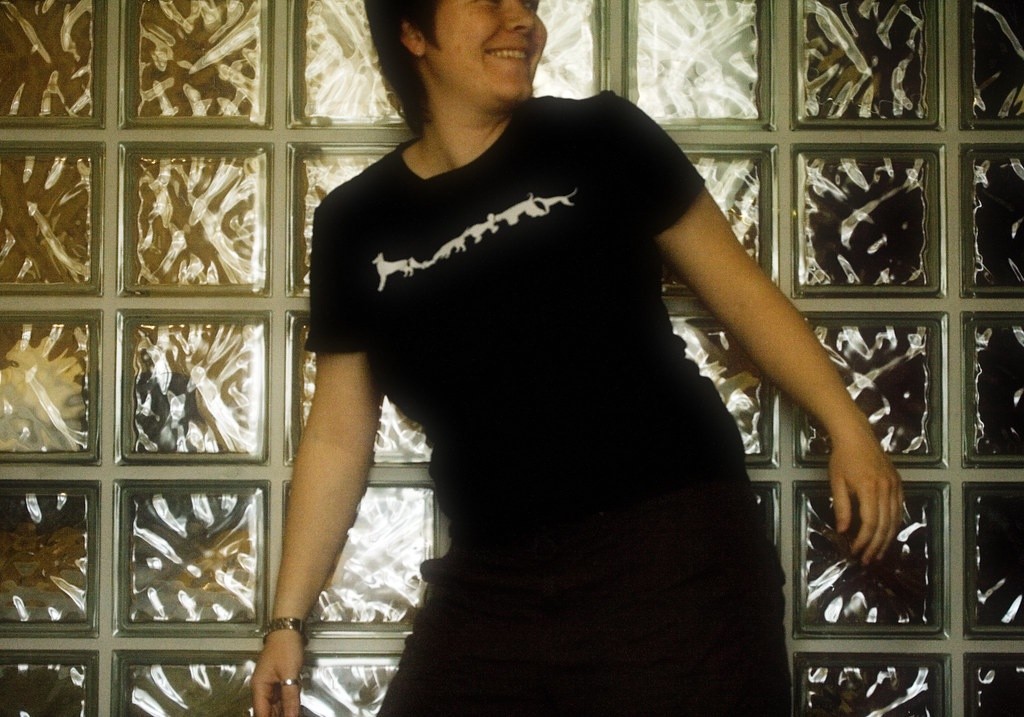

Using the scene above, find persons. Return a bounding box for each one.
[253,0,904,717]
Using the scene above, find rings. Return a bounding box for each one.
[280,679,301,691]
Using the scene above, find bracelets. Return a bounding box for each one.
[260,617,309,647]
